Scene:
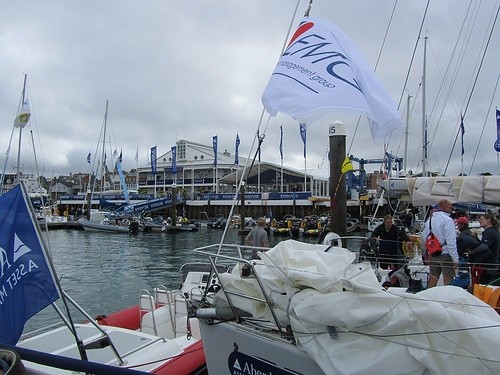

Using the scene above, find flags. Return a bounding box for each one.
[261,15,402,145]
[0,183,60,349]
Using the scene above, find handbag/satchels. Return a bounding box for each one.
[424,233,445,256]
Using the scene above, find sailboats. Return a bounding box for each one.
[184,1,500,375]
[0,74,229,375]
[78,100,129,232]
[36,166,81,228]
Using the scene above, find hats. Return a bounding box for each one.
[454,217,468,223]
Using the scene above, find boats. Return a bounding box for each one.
[143,211,359,238]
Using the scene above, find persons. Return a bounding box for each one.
[422,199,459,288]
[130,219,138,233]
[466,215,500,286]
[245,217,269,259]
[454,217,491,263]
[371,214,410,270]
